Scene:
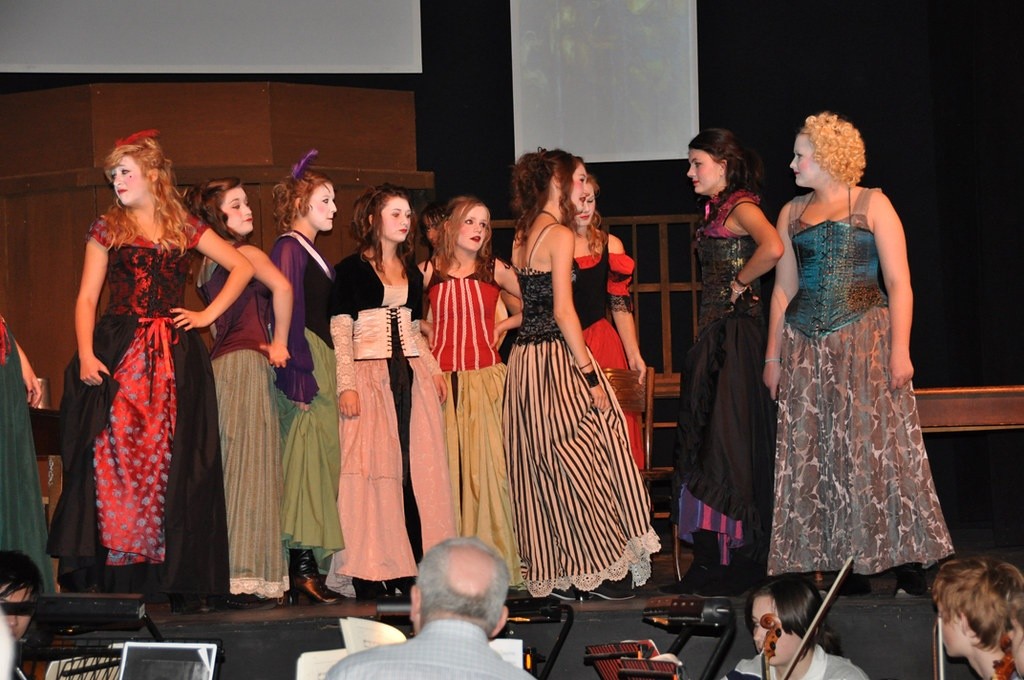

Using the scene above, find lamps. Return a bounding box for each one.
[503,598,574,680]
[642,596,736,680]
[0,596,164,680]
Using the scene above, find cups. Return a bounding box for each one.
[37,378,50,409]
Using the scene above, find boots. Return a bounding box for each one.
[657,531,733,597]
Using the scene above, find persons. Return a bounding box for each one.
[719,574,872,680]
[0,128,661,680]
[931,555,1024,680]
[761,112,956,600]
[655,128,784,598]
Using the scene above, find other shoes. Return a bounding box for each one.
[823,569,872,596]
[217,594,277,610]
[552,588,576,602]
[570,584,636,601]
[168,592,200,614]
[890,564,928,596]
[384,575,415,596]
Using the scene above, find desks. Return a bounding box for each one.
[28,409,78,594]
[817,384,1024,585]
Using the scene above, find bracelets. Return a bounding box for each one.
[581,361,592,369]
[763,358,781,365]
[730,274,747,294]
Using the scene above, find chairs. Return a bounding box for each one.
[602,366,684,584]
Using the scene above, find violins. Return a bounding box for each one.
[760,612,782,680]
[985,630,1016,680]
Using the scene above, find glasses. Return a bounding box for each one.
[364,183,392,213]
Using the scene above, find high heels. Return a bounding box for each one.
[287,548,342,605]
